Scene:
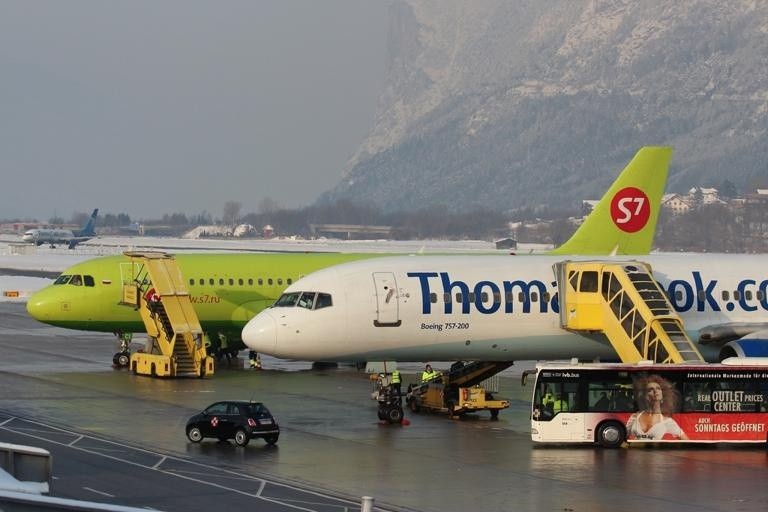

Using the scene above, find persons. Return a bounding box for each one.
[542,388,553,405]
[625,374,690,440]
[553,393,568,415]
[216,332,232,365]
[421,364,440,382]
[390,366,403,394]
[594,391,611,410]
[248,349,259,369]
[203,332,212,356]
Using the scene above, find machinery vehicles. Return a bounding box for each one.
[402,359,514,422]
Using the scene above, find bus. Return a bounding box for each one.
[522,356,768,451]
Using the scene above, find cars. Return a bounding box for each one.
[184,397,280,446]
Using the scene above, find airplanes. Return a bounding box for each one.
[26,143,677,379]
[239,250,768,368]
[22,205,100,250]
